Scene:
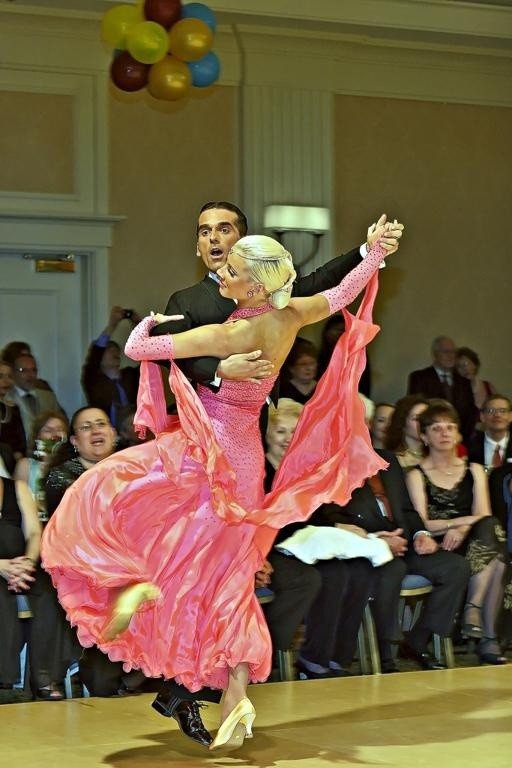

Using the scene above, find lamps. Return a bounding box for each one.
[262,202,330,271]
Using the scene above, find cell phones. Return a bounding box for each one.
[121,309,134,319]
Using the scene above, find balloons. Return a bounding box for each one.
[101,0,220,102]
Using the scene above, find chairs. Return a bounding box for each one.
[11,575,457,701]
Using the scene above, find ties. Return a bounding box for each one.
[493,444,501,466]
[443,374,450,399]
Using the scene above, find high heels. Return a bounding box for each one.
[100,582,167,641]
[210,697,257,748]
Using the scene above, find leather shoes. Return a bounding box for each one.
[295,655,353,680]
[476,635,505,664]
[462,602,484,639]
[29,676,64,700]
[404,641,447,670]
[151,686,214,746]
[382,658,400,673]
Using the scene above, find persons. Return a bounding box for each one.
[147,201,404,747]
[1,307,192,703]
[265,335,512,680]
[39,218,399,752]
